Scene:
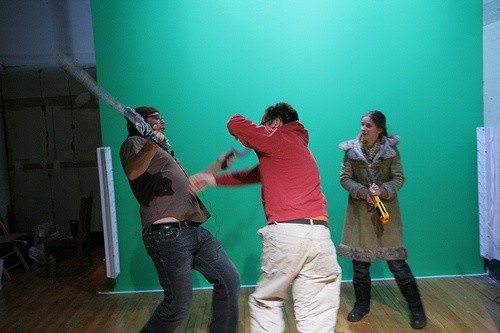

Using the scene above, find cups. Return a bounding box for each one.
[68,219,79,238]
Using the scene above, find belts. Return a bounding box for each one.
[142,219,202,234]
[268,218,329,227]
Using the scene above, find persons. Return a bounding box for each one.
[188,103,341,333]
[120,107,241,333]
[337,111,426,328]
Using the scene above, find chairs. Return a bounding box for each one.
[43,191,94,278]
[0,222,29,280]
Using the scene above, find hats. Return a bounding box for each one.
[128,107,160,133]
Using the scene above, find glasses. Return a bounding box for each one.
[259,118,271,126]
[147,114,159,119]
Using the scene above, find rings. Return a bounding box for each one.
[372,190,375,193]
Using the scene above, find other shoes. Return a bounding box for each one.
[409,300,426,329]
[347,300,370,322]
